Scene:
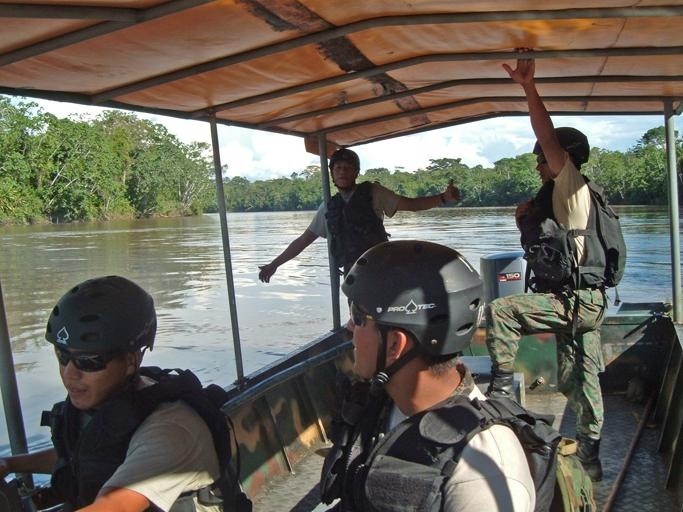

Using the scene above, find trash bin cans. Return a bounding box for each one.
[441,193,447,206]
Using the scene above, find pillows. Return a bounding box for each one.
[533,127,590,165]
[44,275,157,353]
[329,149,360,172]
[341,239,484,356]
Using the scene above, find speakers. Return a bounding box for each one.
[484,367,518,401]
[573,434,603,481]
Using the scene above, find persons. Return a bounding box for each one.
[483,47,606,481]
[0,276,251,512]
[322,240,536,512]
[258,149,463,284]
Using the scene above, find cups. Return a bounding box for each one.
[536,155,546,163]
[347,299,375,327]
[53,346,135,373]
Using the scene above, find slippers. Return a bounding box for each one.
[518,180,626,289]
[487,391,597,512]
